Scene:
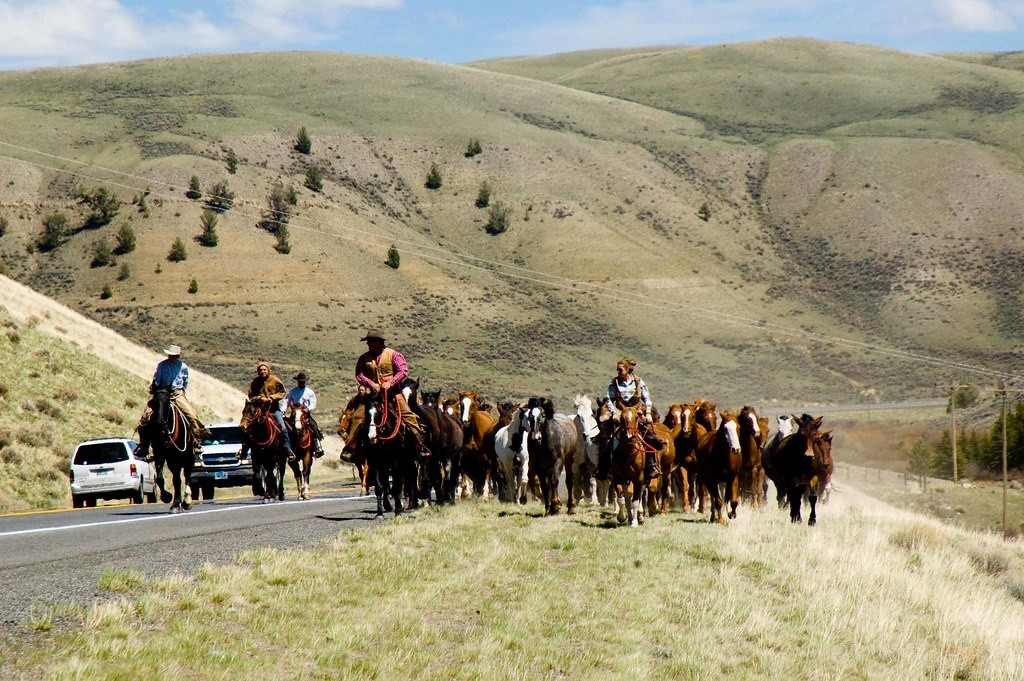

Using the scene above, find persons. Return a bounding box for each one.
[283,368,324,459]
[237,360,294,461]
[356,329,431,460]
[135,344,187,461]
[605,357,663,481]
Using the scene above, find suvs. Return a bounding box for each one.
[187,421,281,501]
[69,437,157,508]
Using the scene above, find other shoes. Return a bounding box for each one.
[194,443,204,456]
[341,450,354,461]
[598,467,607,479]
[135,446,149,457]
[649,465,658,476]
[236,451,247,459]
[287,449,296,459]
[419,447,429,458]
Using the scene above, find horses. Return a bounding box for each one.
[150,379,834,526]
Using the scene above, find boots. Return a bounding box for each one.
[314,438,324,455]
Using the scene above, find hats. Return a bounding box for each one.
[163,345,181,355]
[293,373,310,382]
[360,328,387,342]
